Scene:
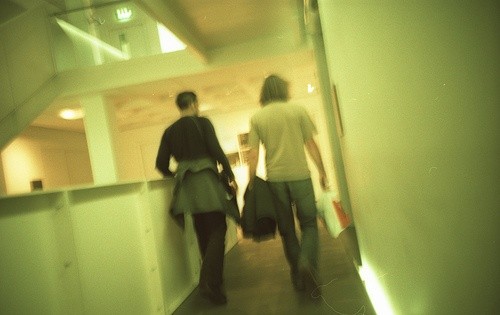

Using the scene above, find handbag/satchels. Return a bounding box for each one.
[316,189,351,237]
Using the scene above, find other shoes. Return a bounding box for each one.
[199,286,228,304]
[303,269,321,298]
[291,273,303,291]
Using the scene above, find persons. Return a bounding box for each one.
[249,75,330,298]
[155,90,238,307]
